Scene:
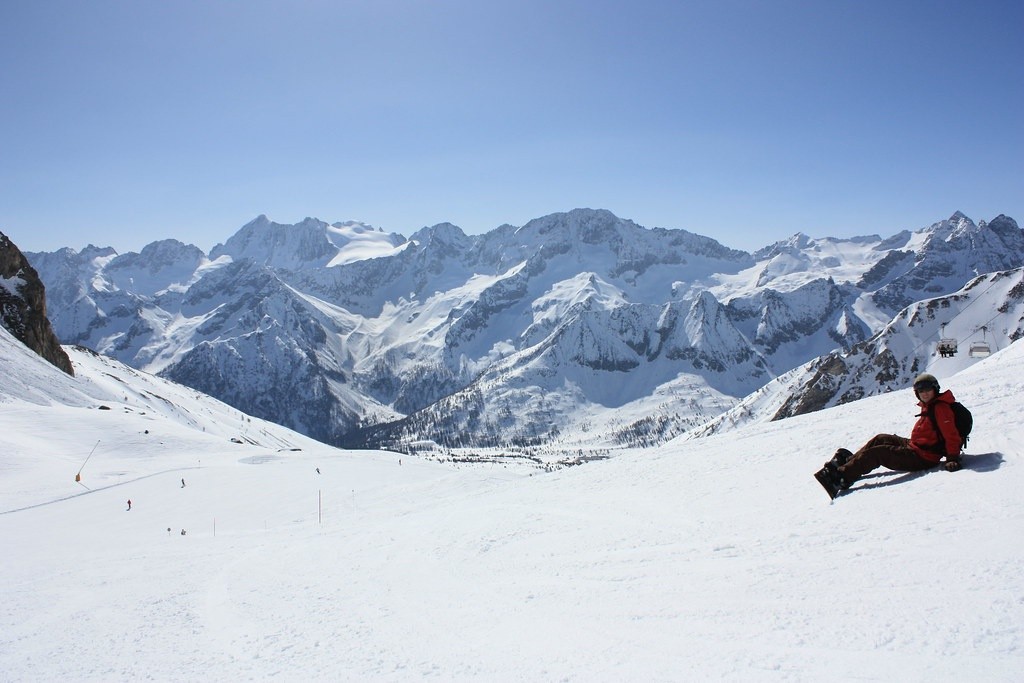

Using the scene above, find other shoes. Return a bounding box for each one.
[825,461,850,490]
[836,453,848,465]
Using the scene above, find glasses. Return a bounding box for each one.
[916,384,933,393]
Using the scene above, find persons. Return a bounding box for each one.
[824,374,962,491]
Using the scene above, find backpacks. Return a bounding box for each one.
[927,399,973,451]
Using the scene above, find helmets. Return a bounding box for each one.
[913,373,940,401]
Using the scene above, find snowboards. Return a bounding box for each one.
[814,448,856,500]
